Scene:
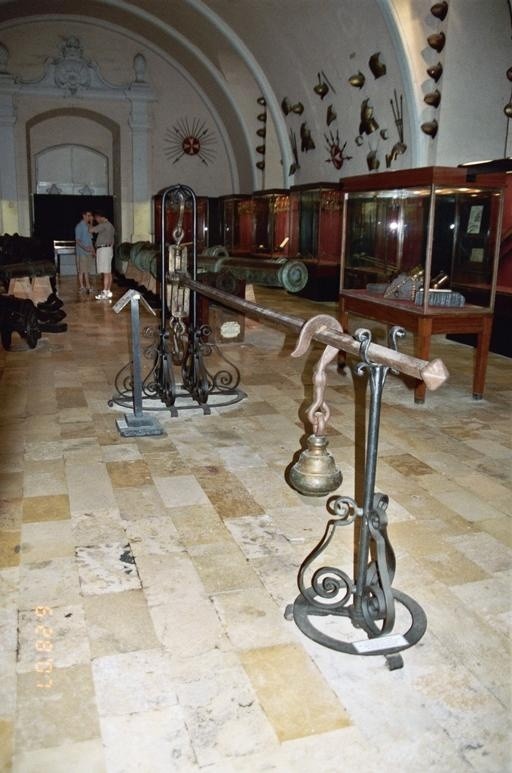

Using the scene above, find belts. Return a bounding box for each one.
[97,244,111,248]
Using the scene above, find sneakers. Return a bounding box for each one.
[79,288,93,294]
[95,291,113,299]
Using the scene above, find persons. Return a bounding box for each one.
[87,211,115,298]
[74,209,96,294]
